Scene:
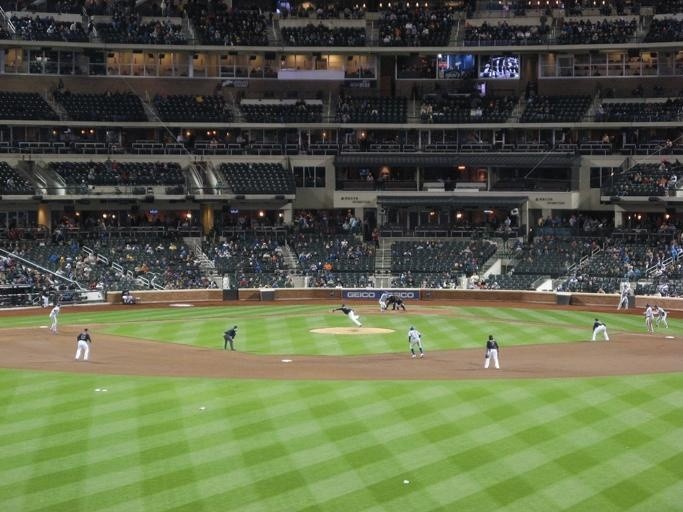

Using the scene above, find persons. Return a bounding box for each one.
[409,326,424,358]
[224,325,238,351]
[75,328,91,361]
[485,335,500,368]
[592,319,609,341]
